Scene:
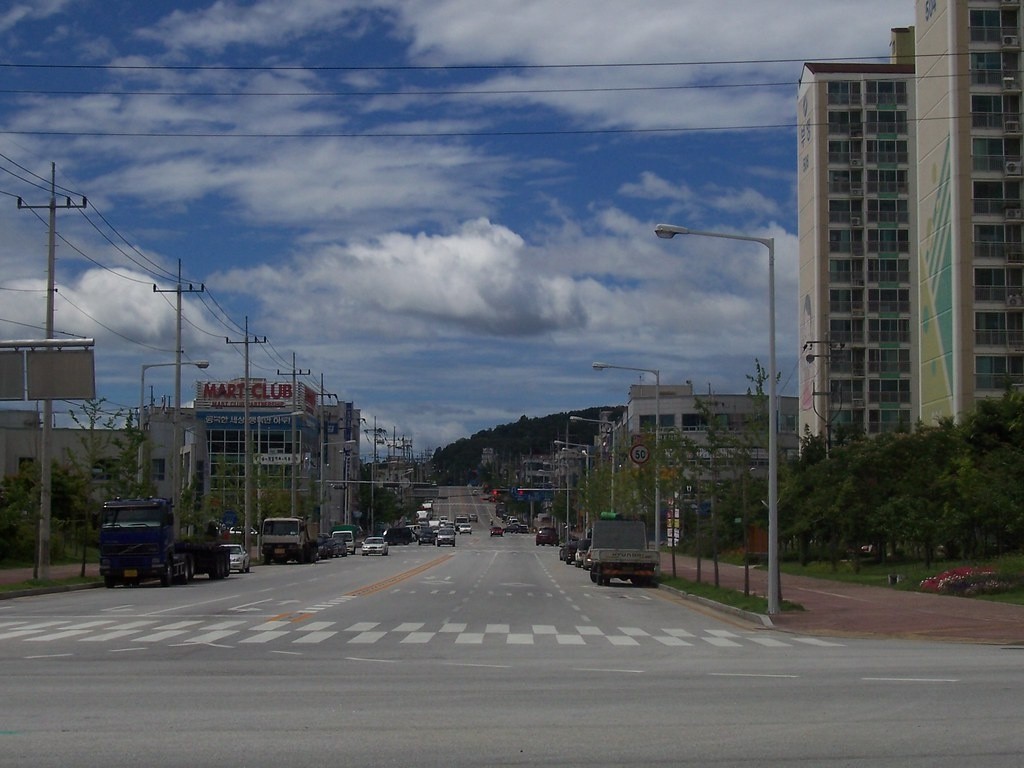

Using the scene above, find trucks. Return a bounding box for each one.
[586,518,659,587]
[332,530,354,554]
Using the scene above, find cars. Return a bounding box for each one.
[361,537,388,556]
[220,544,250,573]
[559,538,593,570]
[489,502,530,536]
[405,499,478,548]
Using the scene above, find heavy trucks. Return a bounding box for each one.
[262,517,332,564]
[99,499,194,588]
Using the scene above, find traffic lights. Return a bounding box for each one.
[493,490,504,494]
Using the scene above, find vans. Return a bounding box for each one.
[383,528,411,546]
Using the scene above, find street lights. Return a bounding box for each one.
[569,415,616,511]
[554,440,589,520]
[657,223,779,613]
[257,410,304,561]
[592,362,661,556]
[138,359,209,497]
[320,440,356,534]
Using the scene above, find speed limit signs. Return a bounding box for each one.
[631,444,649,463]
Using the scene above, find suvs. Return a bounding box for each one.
[536,527,559,546]
